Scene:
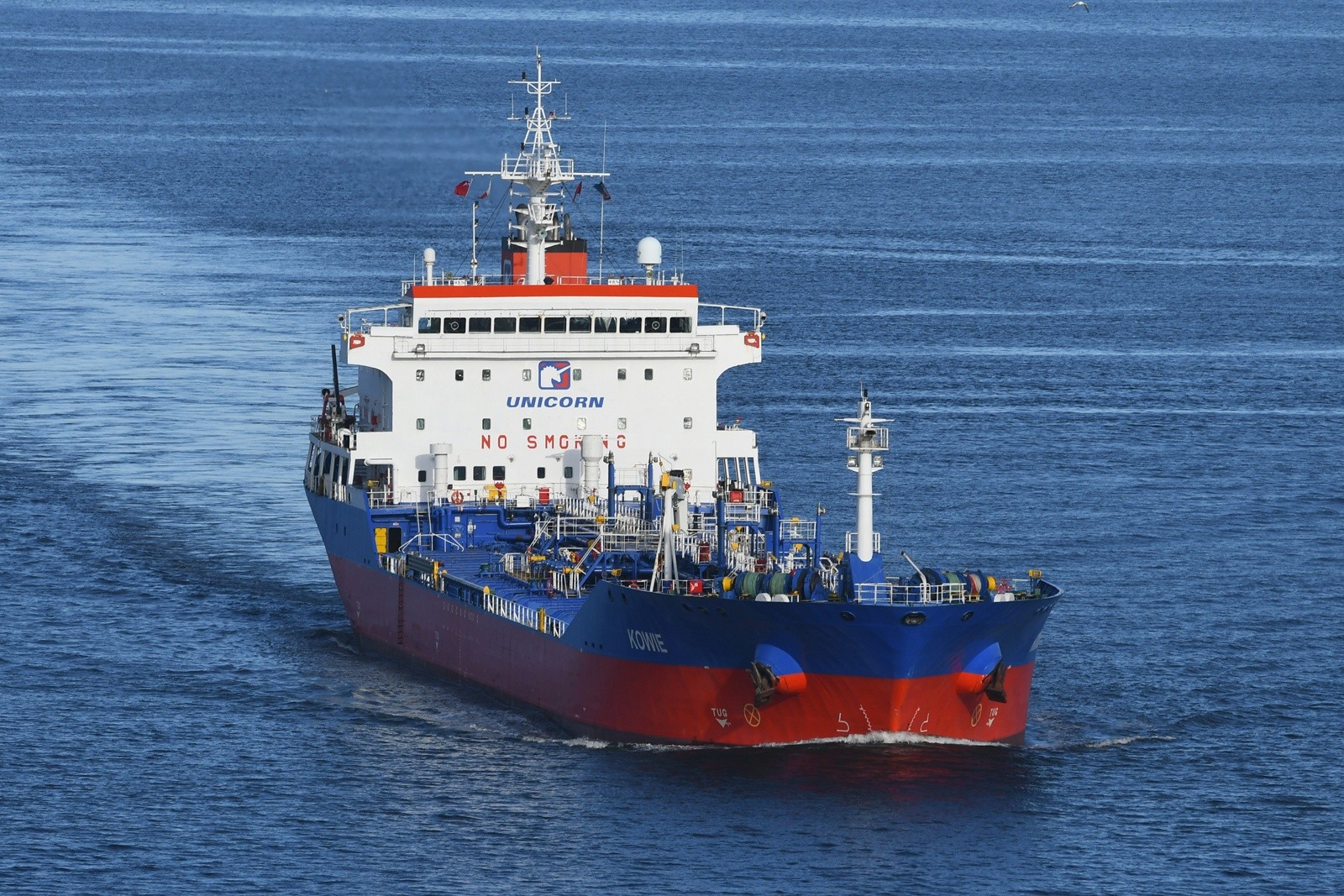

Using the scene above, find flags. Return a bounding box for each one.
[455,179,472,196]
[574,181,582,201]
[479,179,491,199]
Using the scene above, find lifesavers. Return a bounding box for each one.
[451,491,464,504]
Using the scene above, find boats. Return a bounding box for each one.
[301,43,1065,789]
